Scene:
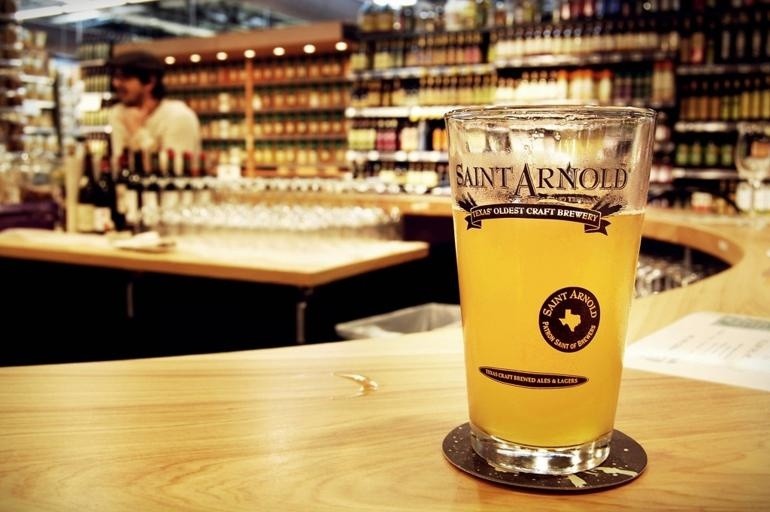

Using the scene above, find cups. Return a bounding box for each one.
[441,103,658,474]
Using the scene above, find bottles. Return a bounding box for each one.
[346,1,770,214]
[72,135,210,236]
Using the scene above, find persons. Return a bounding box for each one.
[92,50,203,182]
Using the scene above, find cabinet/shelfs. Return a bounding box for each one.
[669,56,770,199]
[253,51,349,179]
[350,25,494,184]
[163,59,253,177]
[495,12,669,198]
[0,0,115,187]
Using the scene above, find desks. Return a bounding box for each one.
[2,219,430,345]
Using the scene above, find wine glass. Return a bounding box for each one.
[728,121,770,230]
